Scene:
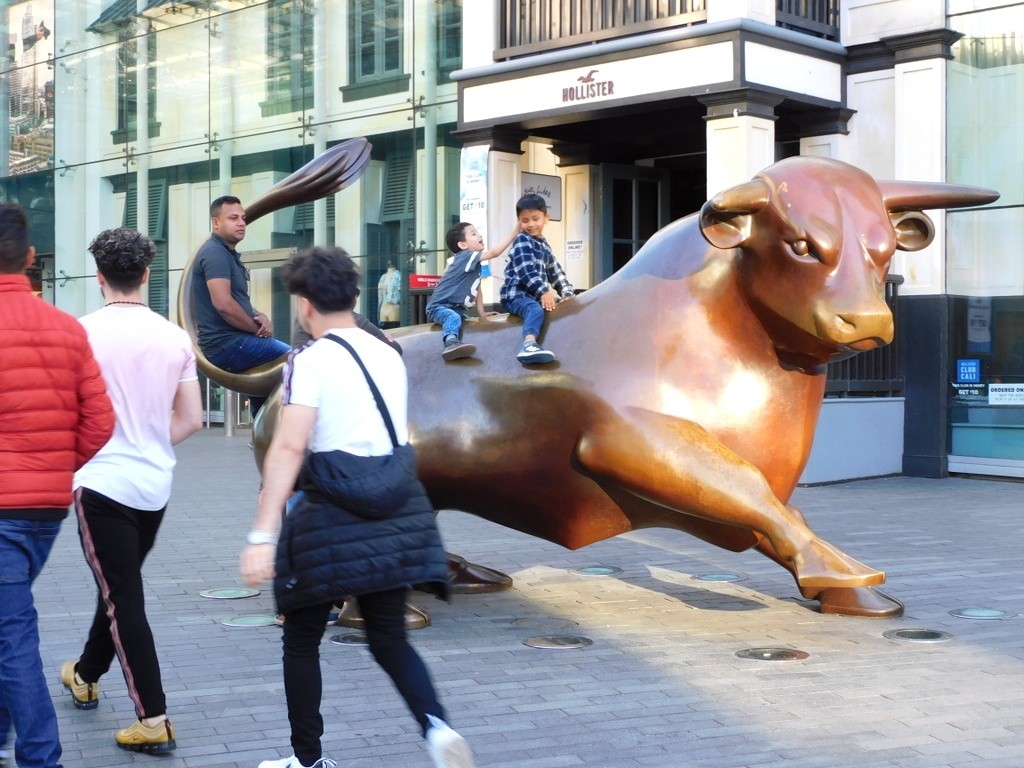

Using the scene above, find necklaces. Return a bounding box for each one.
[105,301,147,308]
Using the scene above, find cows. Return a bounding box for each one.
[176,136,1000,629]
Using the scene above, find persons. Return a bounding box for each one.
[426,222,521,361]
[0,202,116,768]
[61,227,204,754]
[274,309,403,628]
[377,266,401,329]
[191,195,292,449]
[240,244,479,768]
[500,193,589,364]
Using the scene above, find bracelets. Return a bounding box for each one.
[247,531,279,545]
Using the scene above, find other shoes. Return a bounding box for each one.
[257,754,337,768]
[423,713,473,768]
[442,337,476,361]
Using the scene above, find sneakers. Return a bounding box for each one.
[116,718,177,754]
[516,341,555,365]
[61,660,99,709]
